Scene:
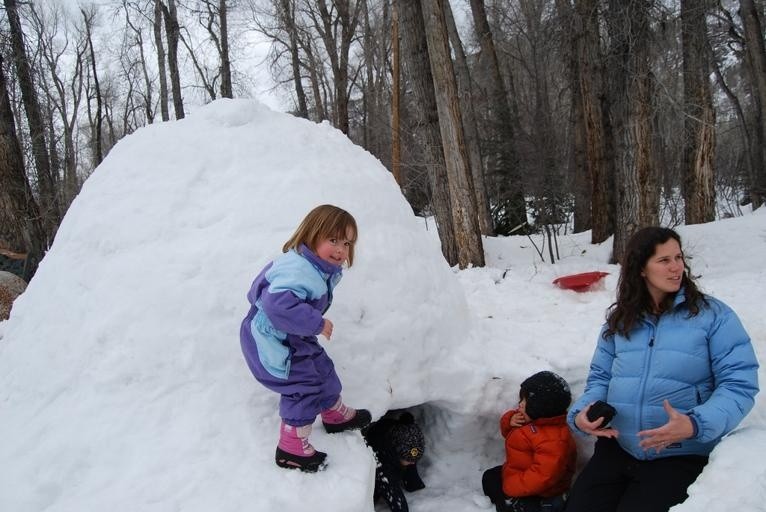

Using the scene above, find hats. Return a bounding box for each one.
[520,371,571,420]
[389,422,425,462]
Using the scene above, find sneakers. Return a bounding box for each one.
[323,409,372,433]
[276,446,328,473]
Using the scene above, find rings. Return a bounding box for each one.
[663,444,665,447]
[661,440,665,444]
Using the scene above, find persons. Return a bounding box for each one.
[480,369,578,511]
[564,225,762,512]
[237,203,373,473]
[362,408,426,512]
